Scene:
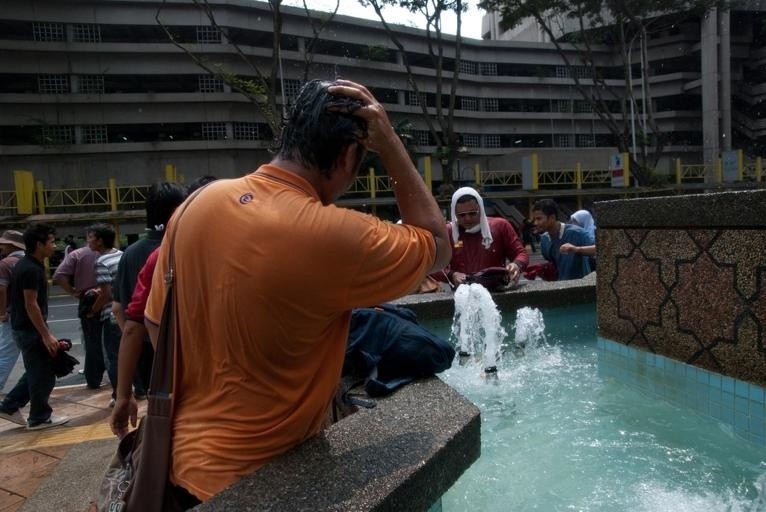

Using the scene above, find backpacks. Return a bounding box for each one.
[337,300,458,398]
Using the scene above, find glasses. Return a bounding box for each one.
[457,207,482,218]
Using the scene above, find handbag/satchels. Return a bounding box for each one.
[418,275,445,294]
[468,263,513,292]
[85,404,177,510]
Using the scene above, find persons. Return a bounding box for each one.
[430,186,595,289]
[0,174,217,428]
[142,74,455,511]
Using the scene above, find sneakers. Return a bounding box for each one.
[29,414,70,431]
[77,368,85,375]
[131,385,147,400]
[108,398,116,406]
[1,409,27,427]
